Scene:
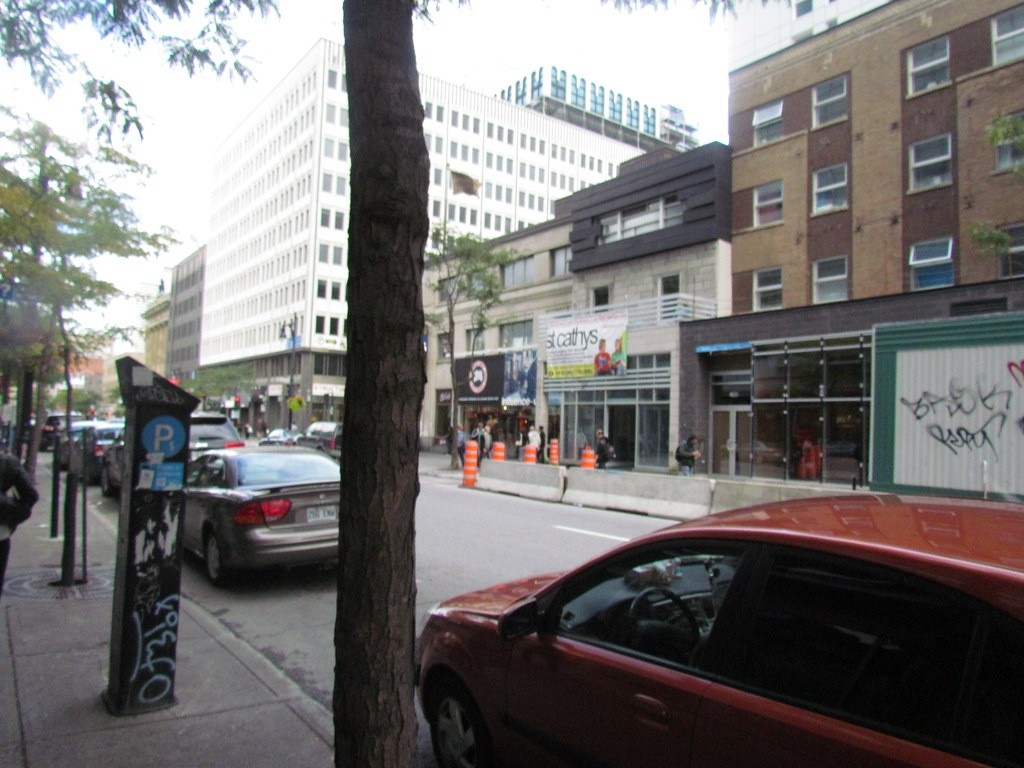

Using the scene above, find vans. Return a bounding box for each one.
[299,418,342,461]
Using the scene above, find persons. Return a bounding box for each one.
[244,424,250,438]
[458,424,464,466]
[291,422,297,430]
[594,339,626,375]
[519,425,545,463]
[0,450,39,594]
[472,423,492,471]
[680,436,700,476]
[596,430,610,468]
[237,419,242,434]
[510,351,536,400]
[444,427,453,454]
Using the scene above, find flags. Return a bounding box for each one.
[451,171,481,198]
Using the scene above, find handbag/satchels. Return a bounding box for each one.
[0,454,32,526]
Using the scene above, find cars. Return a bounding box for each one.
[259,426,303,446]
[415,492,1024,768]
[180,443,340,591]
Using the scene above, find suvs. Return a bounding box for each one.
[24,411,243,499]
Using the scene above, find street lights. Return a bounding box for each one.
[278,313,299,429]
